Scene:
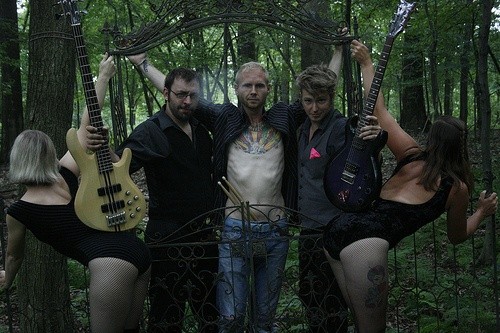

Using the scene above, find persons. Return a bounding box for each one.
[0,53,152,333]
[121,27,352,333]
[84,65,220,332]
[323,40,499,333]
[292,66,380,333]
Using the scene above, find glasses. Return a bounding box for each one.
[168,87,201,99]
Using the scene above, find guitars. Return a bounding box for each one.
[323,0,417,216]
[59,0,148,231]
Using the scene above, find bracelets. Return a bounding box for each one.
[137,57,146,66]
